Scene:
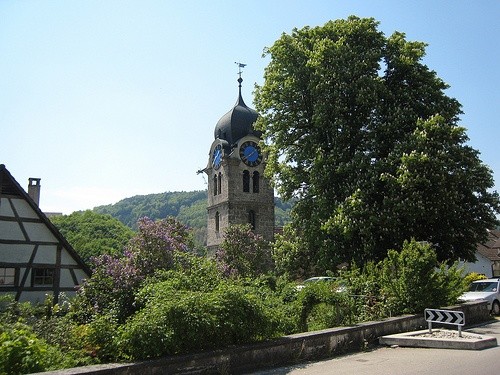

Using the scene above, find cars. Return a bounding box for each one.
[293,276,336,292]
[456,278,499,316]
